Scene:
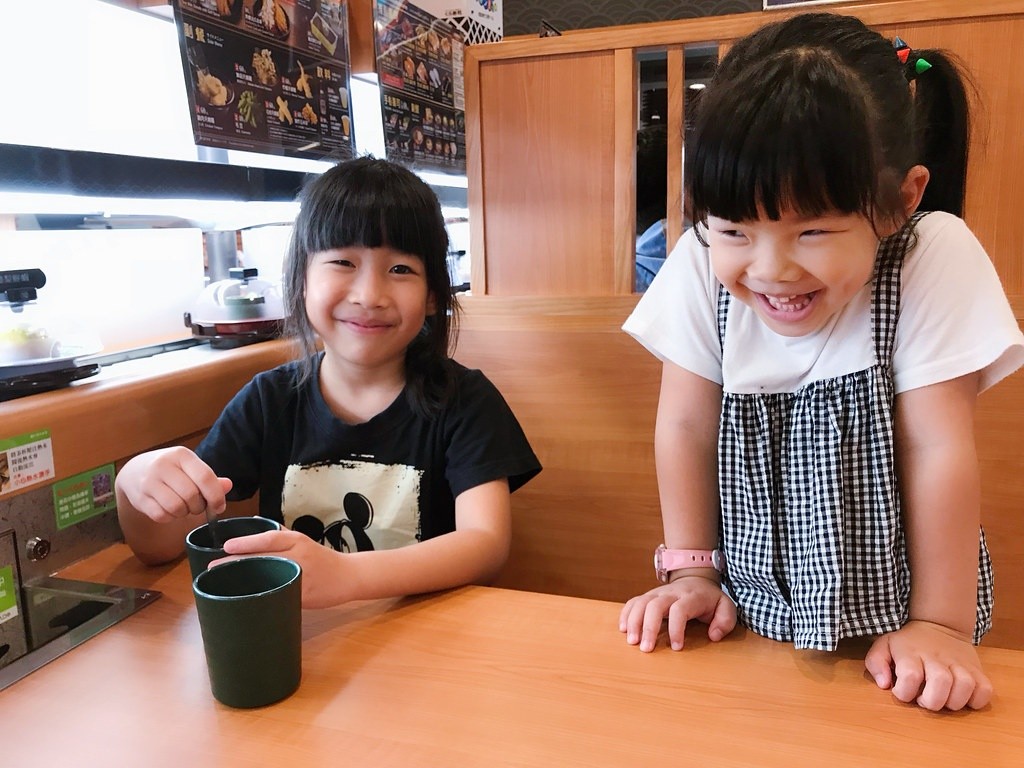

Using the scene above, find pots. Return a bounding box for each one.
[0,286,101,397]
[182,264,295,352]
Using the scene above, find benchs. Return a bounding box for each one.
[445,294,1024,651]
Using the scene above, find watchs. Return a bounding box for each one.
[654,543,725,584]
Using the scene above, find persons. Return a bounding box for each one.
[114,152,544,609]
[634,126,696,294]
[619,13,1024,713]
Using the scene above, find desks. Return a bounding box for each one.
[0,541,1024,768]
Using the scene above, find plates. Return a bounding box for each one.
[378,4,459,158]
[195,68,234,110]
[216,0,244,20]
[252,4,293,39]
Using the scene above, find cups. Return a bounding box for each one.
[339,87,349,109]
[186,516,282,592]
[342,116,351,136]
[192,556,301,708]
